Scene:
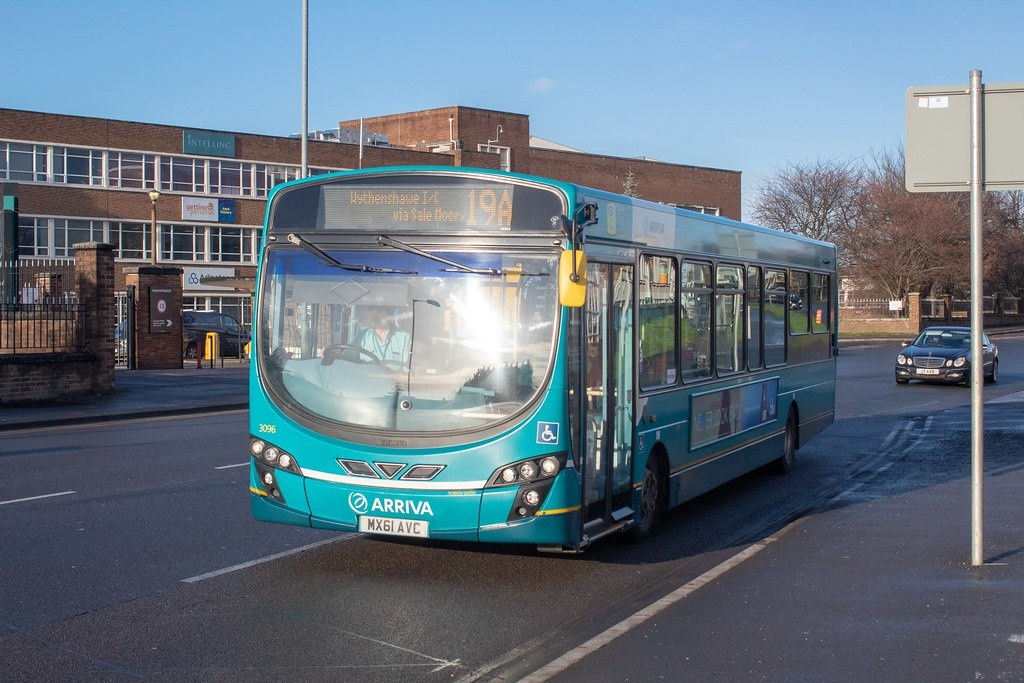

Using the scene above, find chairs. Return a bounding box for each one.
[961,336,971,348]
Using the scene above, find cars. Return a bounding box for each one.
[183,311,252,360]
[895,326,1000,389]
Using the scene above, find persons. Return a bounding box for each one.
[336,305,412,369]
[927,335,943,346]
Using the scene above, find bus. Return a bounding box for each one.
[204,165,840,555]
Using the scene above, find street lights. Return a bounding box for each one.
[147,189,161,265]
